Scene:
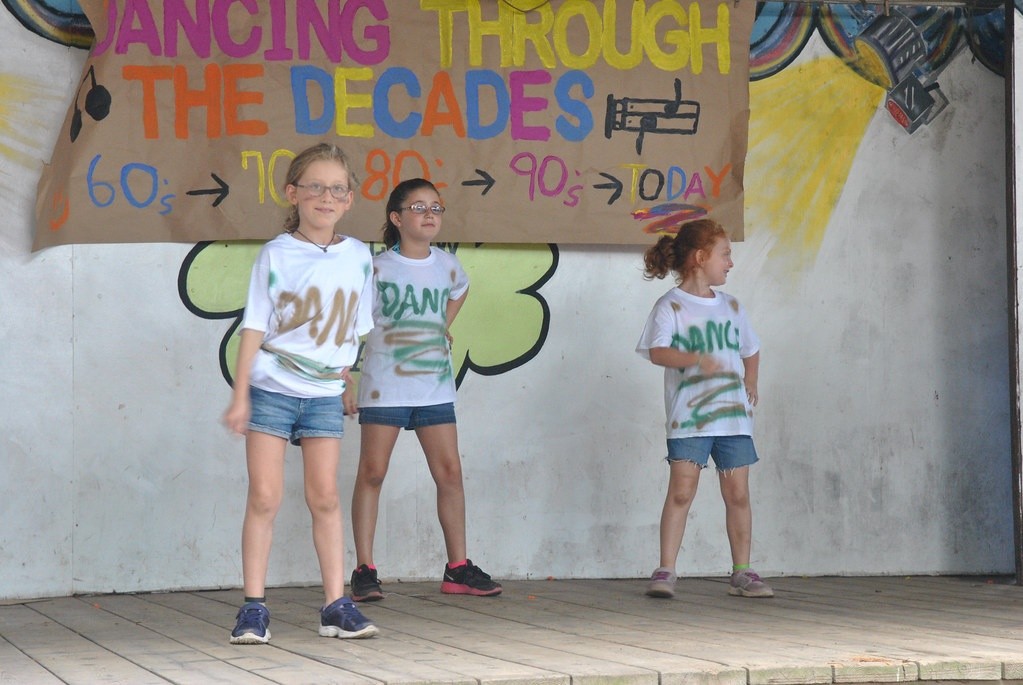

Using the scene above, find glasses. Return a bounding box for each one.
[295,182,349,199]
[397,202,446,214]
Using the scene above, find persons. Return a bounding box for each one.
[222,143,382,642]
[349,177,503,601]
[635,220,774,598]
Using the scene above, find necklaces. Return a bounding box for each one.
[295,229,335,253]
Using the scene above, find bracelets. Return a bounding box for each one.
[697,354,703,368]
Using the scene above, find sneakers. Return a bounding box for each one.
[229,601,271,643]
[646,568,677,597]
[319,597,379,639]
[729,569,772,597]
[351,564,384,602]
[440,559,503,595]
